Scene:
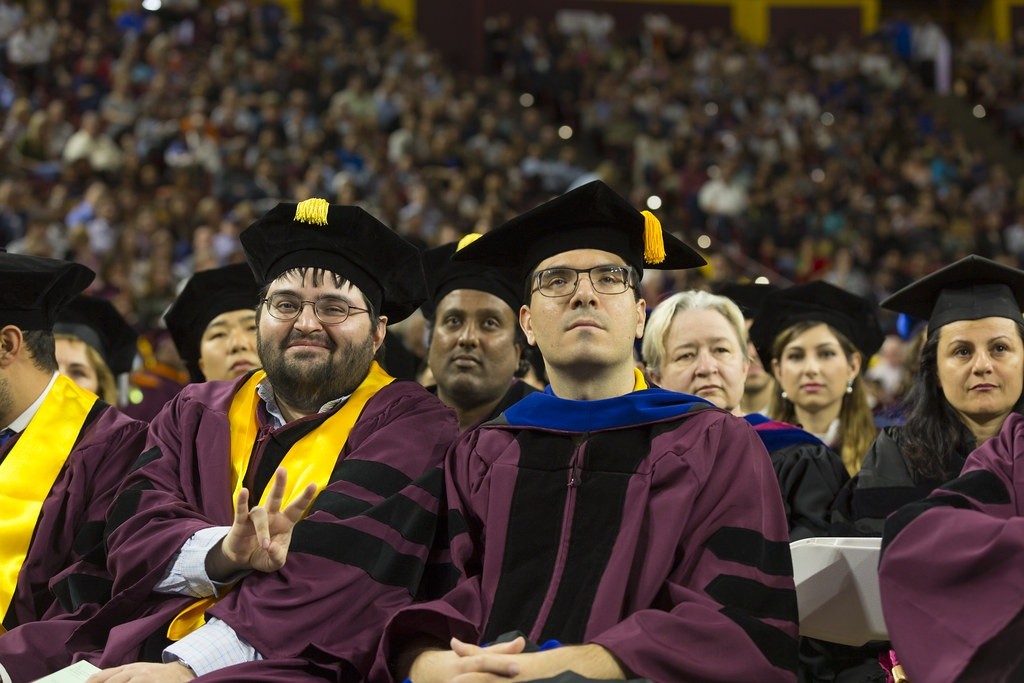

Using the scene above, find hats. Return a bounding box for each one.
[747,280,886,380]
[163,262,261,362]
[0,248,95,328]
[239,198,430,325]
[878,255,1024,337]
[47,295,137,377]
[418,233,524,322]
[450,180,707,298]
[710,284,784,320]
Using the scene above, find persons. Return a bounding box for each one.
[879,397,1024,683]
[834,255,1024,683]
[642,291,848,682]
[52,298,135,409]
[65,199,457,683]
[0,0,1024,416]
[748,279,892,478]
[0,253,149,682]
[421,233,542,435]
[370,184,799,683]
[165,263,263,381]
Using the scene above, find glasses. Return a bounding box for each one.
[258,292,376,324]
[529,265,641,298]
